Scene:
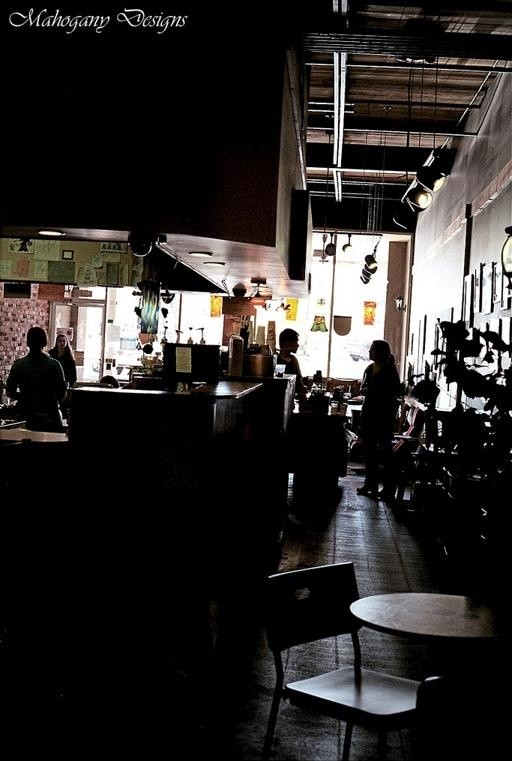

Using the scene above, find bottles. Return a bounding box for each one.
[247,321,254,347]
[229,332,244,375]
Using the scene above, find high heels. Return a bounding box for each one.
[374,485,396,497]
[356,482,379,496]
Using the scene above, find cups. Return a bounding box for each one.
[266,321,275,352]
[256,325,265,345]
[340,404,346,415]
[276,364,285,377]
[331,400,338,414]
[344,392,351,398]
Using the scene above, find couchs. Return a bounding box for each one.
[299,376,362,412]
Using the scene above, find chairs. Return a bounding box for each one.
[264,561,426,761]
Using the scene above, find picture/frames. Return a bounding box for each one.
[492,262,503,303]
[105,262,119,285]
[463,273,473,322]
[500,316,512,357]
[481,261,495,316]
[500,273,510,309]
[62,250,73,260]
[473,263,481,313]
[488,320,501,348]
[479,322,488,345]
[92,255,102,268]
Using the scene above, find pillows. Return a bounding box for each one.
[325,377,357,393]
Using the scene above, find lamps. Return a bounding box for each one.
[414,166,446,193]
[342,235,353,253]
[393,216,414,231]
[324,233,336,256]
[360,255,378,285]
[405,188,432,213]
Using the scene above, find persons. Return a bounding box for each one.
[357,341,400,496]
[276,330,306,398]
[6,328,66,431]
[48,335,76,387]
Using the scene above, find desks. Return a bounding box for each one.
[352,593,498,639]
